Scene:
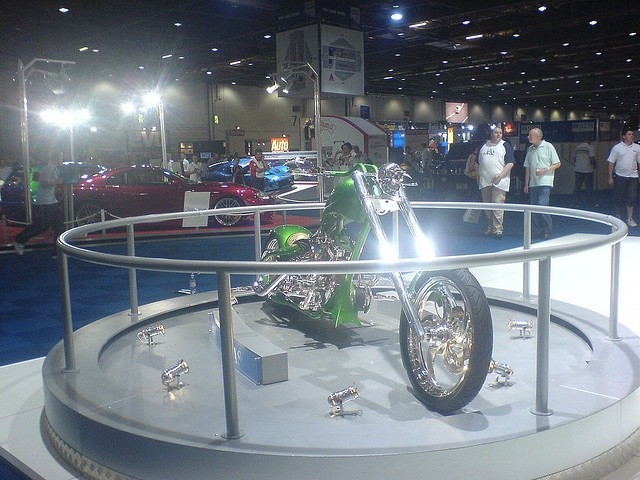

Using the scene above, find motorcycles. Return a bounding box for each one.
[251,160,494,415]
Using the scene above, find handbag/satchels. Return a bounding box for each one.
[462,209,481,224]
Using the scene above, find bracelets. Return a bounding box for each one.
[547,166,551,172]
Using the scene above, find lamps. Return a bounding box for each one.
[160,360,189,389]
[488,359,511,389]
[324,383,360,417]
[263,58,322,182]
[389,8,404,23]
[137,323,166,345]
[231,295,237,304]
[507,317,534,337]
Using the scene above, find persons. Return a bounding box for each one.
[176,152,189,171]
[230,156,246,185]
[570,135,600,209]
[334,142,353,165]
[475,125,516,240]
[186,153,204,181]
[11,147,68,259]
[522,127,562,239]
[249,148,270,191]
[397,141,435,191]
[606,127,640,228]
[207,149,231,165]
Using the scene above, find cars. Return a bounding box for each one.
[282,156,318,182]
[0,161,119,227]
[199,158,296,195]
[408,149,446,187]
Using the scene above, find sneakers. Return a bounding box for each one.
[492,232,503,239]
[542,229,550,238]
[532,229,542,238]
[626,218,637,227]
[13,241,23,255]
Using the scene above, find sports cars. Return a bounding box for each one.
[54,164,274,232]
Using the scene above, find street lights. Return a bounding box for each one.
[120,88,170,183]
[36,104,93,162]
[266,63,325,220]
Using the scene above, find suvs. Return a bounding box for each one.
[436,138,516,201]
